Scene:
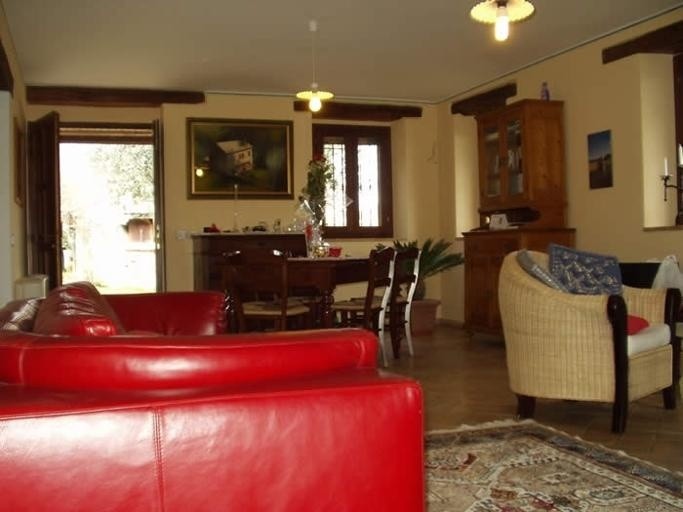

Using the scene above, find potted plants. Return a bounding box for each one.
[376,238,466,338]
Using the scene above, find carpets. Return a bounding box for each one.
[426,418,683,512]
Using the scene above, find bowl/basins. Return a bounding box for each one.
[329,247,342,257]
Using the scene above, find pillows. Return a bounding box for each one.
[549,243,623,295]
[514,248,569,293]
[626,313,649,335]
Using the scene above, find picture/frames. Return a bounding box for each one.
[186,117,294,200]
[588,129,612,187]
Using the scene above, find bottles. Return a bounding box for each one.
[487,149,520,197]
[319,243,326,258]
[312,229,319,258]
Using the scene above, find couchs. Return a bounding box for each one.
[0,283,426,512]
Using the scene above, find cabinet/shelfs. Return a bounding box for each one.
[475,98,566,229]
[462,229,575,338]
[192,233,307,334]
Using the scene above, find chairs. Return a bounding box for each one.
[331,248,423,368]
[207,247,311,332]
[498,250,679,432]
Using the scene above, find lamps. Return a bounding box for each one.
[295,19,334,113]
[468,0,536,44]
